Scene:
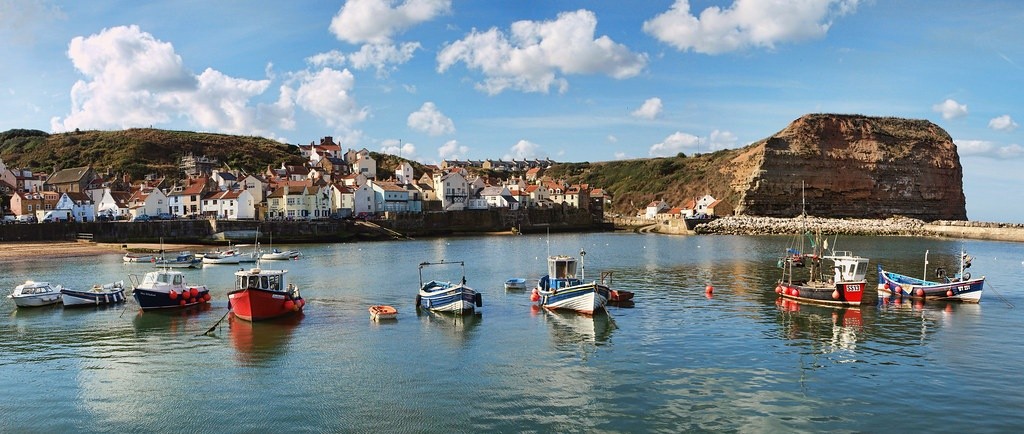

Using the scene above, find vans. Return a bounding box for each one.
[41,210,72,223]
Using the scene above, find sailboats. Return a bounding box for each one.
[776,180,816,283]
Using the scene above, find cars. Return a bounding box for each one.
[159,213,172,220]
[134,214,150,222]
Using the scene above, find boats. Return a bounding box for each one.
[133,239,211,310]
[877,232,986,305]
[195,249,300,263]
[369,306,397,321]
[155,250,204,269]
[227,269,305,322]
[123,254,153,263]
[7,282,62,307]
[775,224,870,306]
[531,249,611,316]
[418,262,481,316]
[611,289,635,301]
[60,280,126,307]
[505,278,526,289]
